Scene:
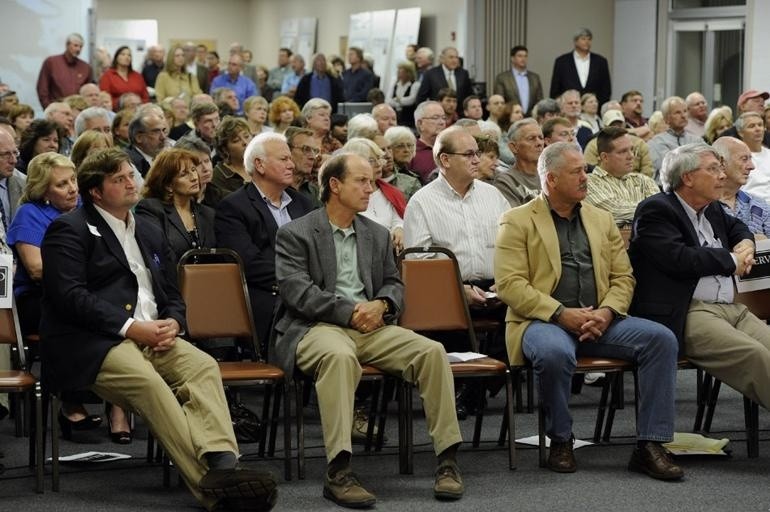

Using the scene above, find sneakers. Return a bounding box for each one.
[323,465,376,508]
[584,372,606,385]
[211,488,278,512]
[198,467,280,499]
[351,408,379,441]
[433,459,464,499]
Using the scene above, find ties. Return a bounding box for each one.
[447,71,453,90]
[595,118,602,131]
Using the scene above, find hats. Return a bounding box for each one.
[602,109,626,127]
[737,89,770,107]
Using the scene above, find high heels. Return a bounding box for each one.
[105,399,132,444]
[57,407,103,440]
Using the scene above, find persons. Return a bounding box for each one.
[2,25,770,512]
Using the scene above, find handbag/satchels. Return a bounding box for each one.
[227,398,261,444]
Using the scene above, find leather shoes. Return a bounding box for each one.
[454,391,469,421]
[465,389,488,416]
[547,432,577,472]
[628,441,684,479]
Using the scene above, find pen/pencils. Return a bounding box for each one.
[470,283,487,307]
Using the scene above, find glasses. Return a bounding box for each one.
[446,150,482,160]
[293,145,320,156]
[422,115,448,122]
[697,161,728,173]
[141,129,169,137]
[0,150,20,160]
[393,143,414,151]
